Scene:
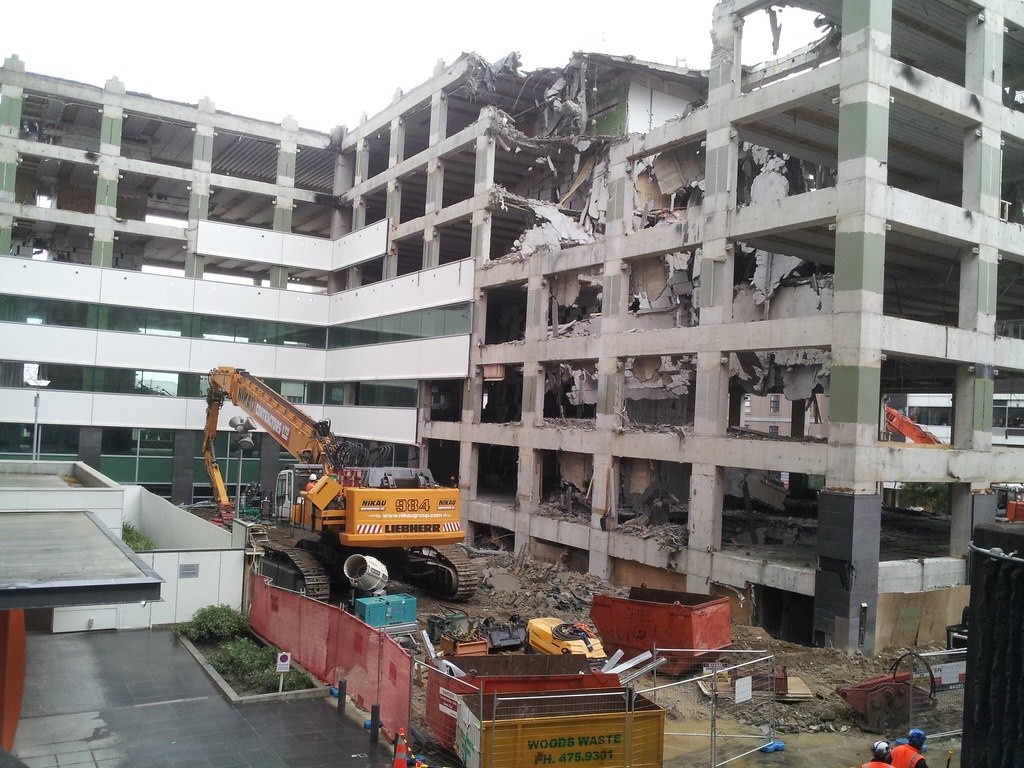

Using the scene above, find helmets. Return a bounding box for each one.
[908,728,927,750]
[871,741,889,760]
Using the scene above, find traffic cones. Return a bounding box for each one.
[392,728,407,768]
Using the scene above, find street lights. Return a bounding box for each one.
[24,377,51,461]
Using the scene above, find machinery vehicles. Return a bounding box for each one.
[200,365,480,605]
[885,405,1024,523]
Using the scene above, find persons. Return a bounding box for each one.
[306,474,317,492]
[861,741,895,768]
[888,728,928,768]
